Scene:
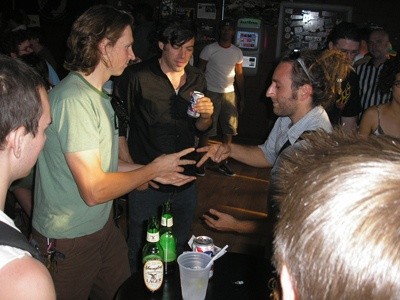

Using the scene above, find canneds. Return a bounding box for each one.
[186,91,204,118]
[192,235,214,279]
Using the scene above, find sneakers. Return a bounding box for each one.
[219,164,236,176]
[195,167,206,177]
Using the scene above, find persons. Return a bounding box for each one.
[271,125,400,300]
[112,16,213,275]
[0,3,158,257]
[195,19,244,177]
[32,4,196,300]
[0,54,56,300]
[304,23,400,138]
[196,50,335,278]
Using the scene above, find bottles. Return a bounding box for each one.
[160,200,177,278]
[141,216,164,300]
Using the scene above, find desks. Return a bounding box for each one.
[114,252,278,300]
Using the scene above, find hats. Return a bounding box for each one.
[222,18,233,27]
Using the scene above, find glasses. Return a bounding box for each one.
[111,93,127,129]
[289,48,314,82]
[334,44,360,56]
[17,43,34,53]
[394,83,400,87]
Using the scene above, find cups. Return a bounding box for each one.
[177,252,214,300]
